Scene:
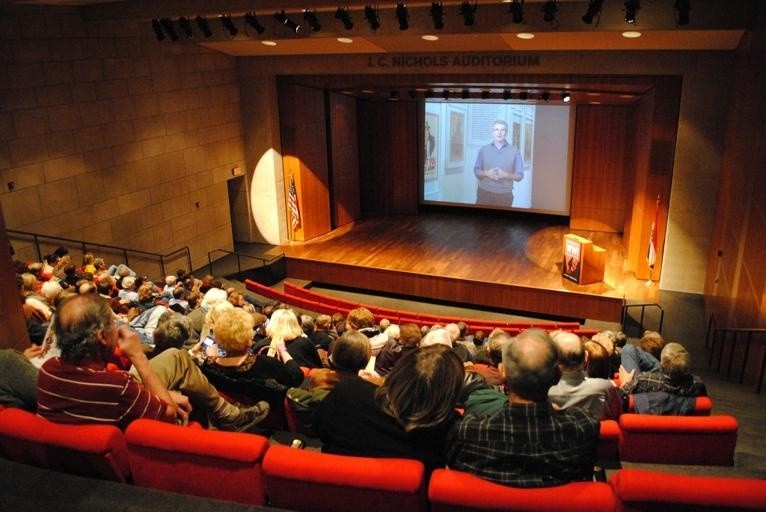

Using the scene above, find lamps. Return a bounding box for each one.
[147,0,693,44]
[351,85,574,104]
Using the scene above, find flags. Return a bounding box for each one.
[287,176,300,231]
[646,196,661,272]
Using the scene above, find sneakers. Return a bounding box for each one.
[218,399,271,434]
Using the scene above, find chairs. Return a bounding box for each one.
[245,279,598,337]
[1,371,766,512]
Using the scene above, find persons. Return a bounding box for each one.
[473,120,524,207]
[424,121,435,173]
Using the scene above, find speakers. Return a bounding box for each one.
[650,141,673,178]
